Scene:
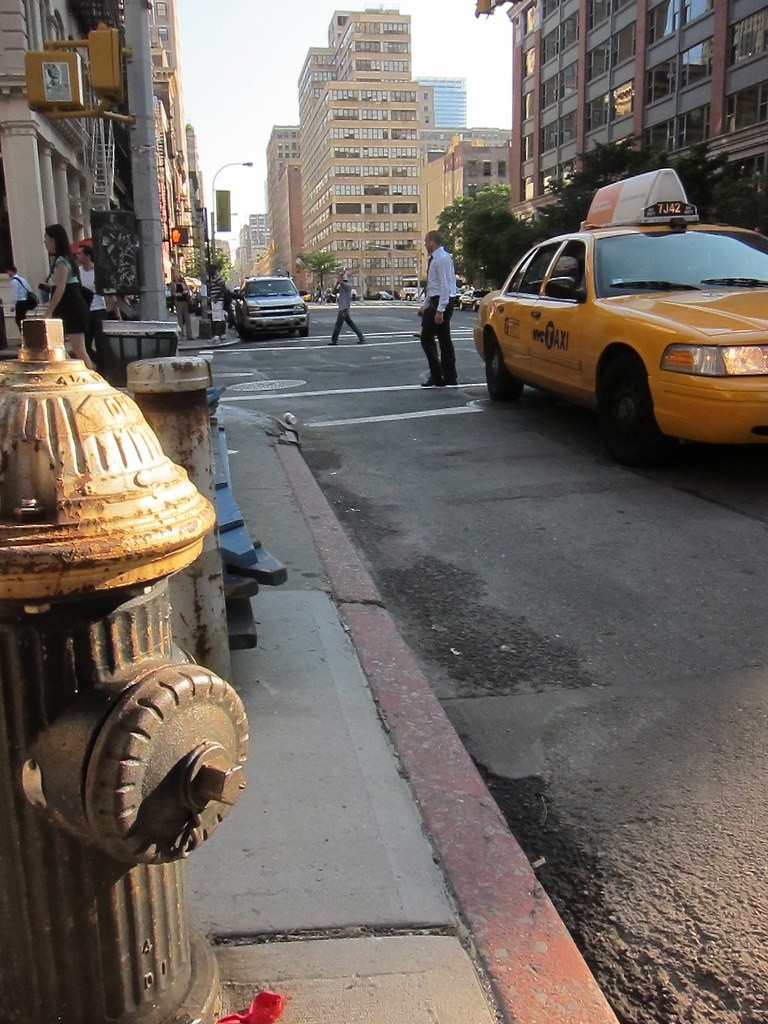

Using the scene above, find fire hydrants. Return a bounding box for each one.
[0,315,253,1024]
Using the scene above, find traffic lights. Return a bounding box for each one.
[172,228,188,246]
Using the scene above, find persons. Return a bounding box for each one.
[326,267,368,347]
[417,230,460,388]
[40,222,94,373]
[6,262,34,347]
[204,265,228,346]
[70,234,174,370]
[186,277,250,330]
[171,268,194,342]
[307,281,344,306]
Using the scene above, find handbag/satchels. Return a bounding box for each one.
[11,276,40,310]
[77,275,94,311]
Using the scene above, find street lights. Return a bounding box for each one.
[211,161,253,265]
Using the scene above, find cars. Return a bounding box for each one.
[473,168,768,454]
[294,275,492,311]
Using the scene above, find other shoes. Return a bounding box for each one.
[421,372,459,387]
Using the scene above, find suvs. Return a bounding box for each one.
[236,275,310,340]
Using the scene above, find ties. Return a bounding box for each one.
[425,256,434,298]
